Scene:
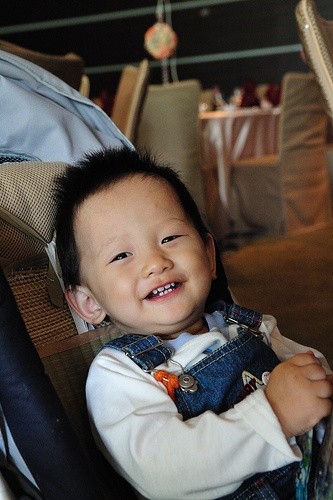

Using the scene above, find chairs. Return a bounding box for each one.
[110,58,147,141]
[229,71,330,239]
[77,75,89,98]
[294,0,333,121]
[133,80,205,219]
[1,41,81,93]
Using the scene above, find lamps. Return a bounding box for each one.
[143,0,176,58]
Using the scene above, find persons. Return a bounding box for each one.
[49,145,333,500]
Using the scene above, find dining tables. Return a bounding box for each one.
[199,106,285,240]
